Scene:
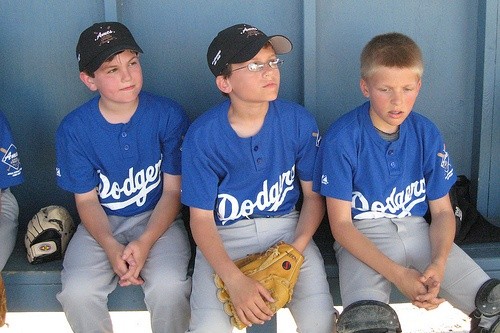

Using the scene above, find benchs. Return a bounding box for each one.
[0,212,500,312]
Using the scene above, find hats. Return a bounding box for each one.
[206,23,294,76]
[75,21,145,74]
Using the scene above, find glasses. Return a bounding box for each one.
[225,58,284,77]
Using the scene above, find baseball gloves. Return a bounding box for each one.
[214,239,305,330]
[24,205,75,264]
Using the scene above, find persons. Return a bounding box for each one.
[0,104,25,330]
[312,31,500,333]
[181,24,338,333]
[54,20,191,333]
